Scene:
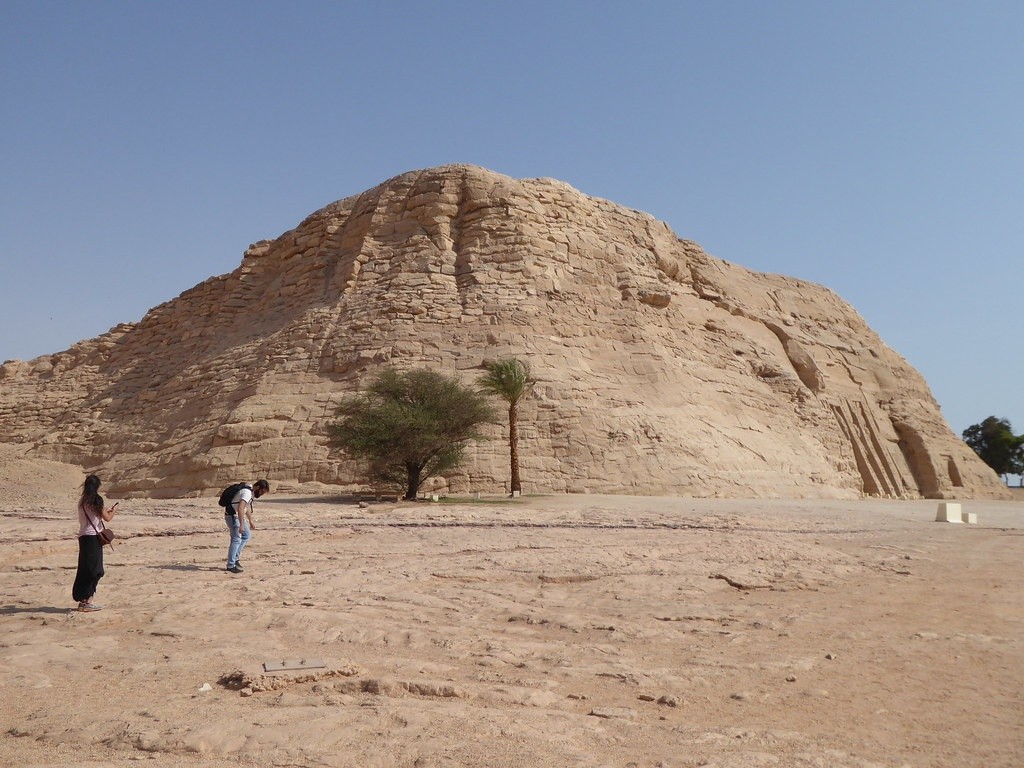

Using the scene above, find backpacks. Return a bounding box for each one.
[218,482,253,514]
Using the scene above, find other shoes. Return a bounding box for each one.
[235,561,243,568]
[227,566,243,573]
[78,602,103,612]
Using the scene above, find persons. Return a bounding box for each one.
[219,480,270,573]
[72,474,120,612]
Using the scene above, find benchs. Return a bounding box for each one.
[353,491,405,501]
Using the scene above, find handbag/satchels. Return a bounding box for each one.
[98,528,114,545]
